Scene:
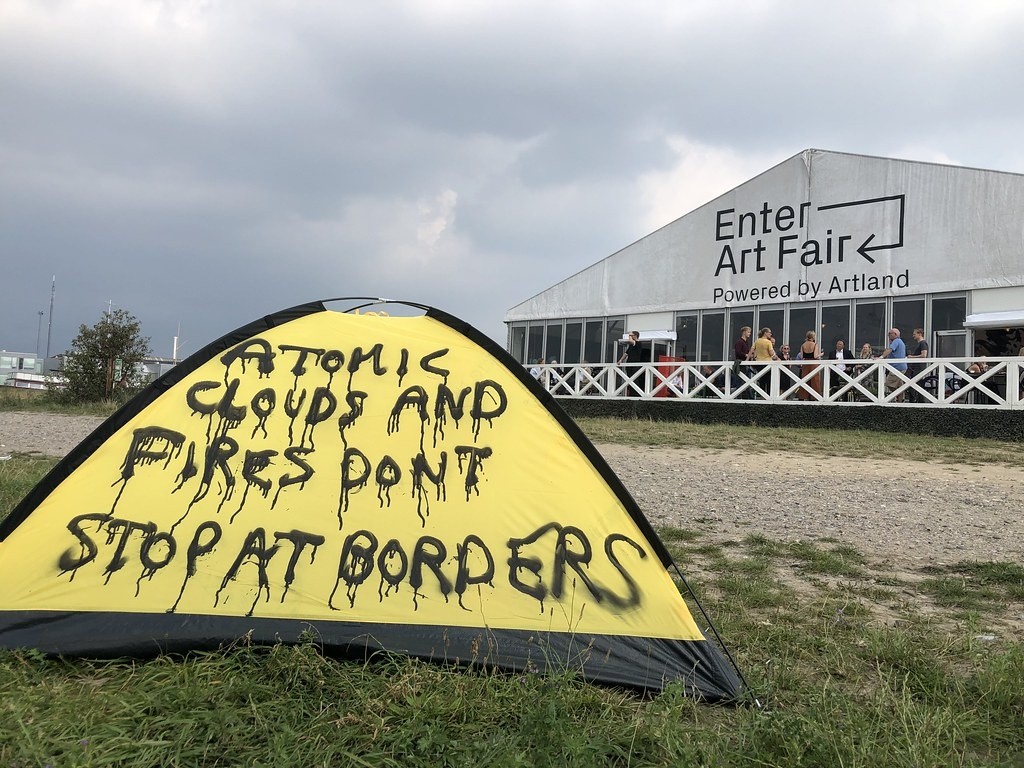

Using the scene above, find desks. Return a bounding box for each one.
[992,374,1005,385]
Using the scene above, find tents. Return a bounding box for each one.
[0,297,761,710]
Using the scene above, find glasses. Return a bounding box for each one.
[783,348,789,350]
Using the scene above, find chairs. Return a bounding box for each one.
[952,373,985,404]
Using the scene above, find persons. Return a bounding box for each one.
[530,358,565,395]
[796,331,824,401]
[769,337,777,360]
[777,345,792,391]
[617,331,641,396]
[961,356,1000,404]
[745,328,781,396]
[735,326,753,399]
[857,343,877,401]
[579,361,590,383]
[879,328,908,403]
[665,370,683,396]
[699,366,718,395]
[908,329,928,403]
[829,339,855,402]
